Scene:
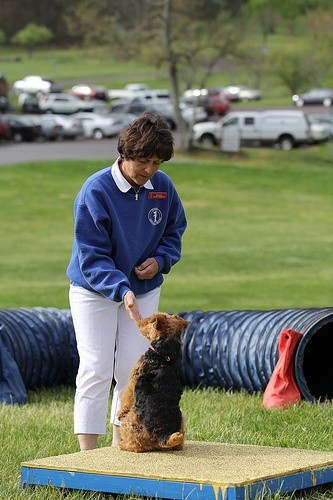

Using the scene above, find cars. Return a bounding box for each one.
[0,75,263,144]
[308,113,333,143]
[292,86,333,108]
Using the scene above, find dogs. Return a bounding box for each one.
[118,312,190,453]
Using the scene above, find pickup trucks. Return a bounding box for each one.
[192,108,312,150]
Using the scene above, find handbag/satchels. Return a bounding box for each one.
[262,328,303,414]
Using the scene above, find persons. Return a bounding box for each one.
[65,116,187,452]
